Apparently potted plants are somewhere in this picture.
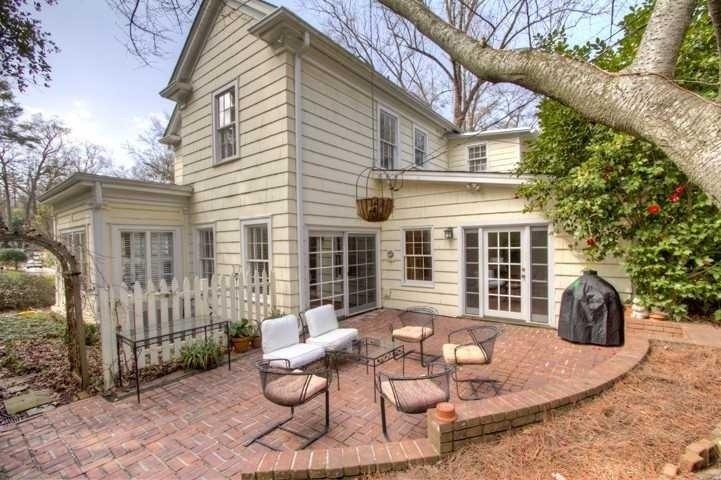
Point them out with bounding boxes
[177,335,223,372]
[229,318,264,352]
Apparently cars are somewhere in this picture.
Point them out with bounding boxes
[25,252,42,269]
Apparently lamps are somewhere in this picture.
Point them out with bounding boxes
[443,228,454,242]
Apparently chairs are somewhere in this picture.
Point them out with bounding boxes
[441,325,499,401]
[388,305,441,367]
[242,357,329,452]
[255,303,361,391]
[377,368,452,433]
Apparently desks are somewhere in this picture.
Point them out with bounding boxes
[116,315,232,402]
